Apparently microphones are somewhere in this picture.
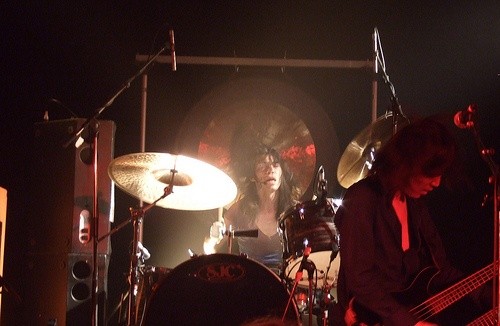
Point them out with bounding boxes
[365,30,378,74]
[295,247,311,281]
[169,29,176,71]
[454,103,477,128]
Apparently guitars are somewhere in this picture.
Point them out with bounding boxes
[347,262,500,326]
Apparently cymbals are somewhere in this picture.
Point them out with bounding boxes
[337,110,395,190]
[107,152,238,210]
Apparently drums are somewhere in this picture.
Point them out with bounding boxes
[279,197,342,288]
[136,266,175,326]
[150,252,302,326]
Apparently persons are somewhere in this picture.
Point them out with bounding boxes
[333,121,492,326]
[203,148,305,277]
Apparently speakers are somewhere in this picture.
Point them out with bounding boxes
[18,120,114,326]
